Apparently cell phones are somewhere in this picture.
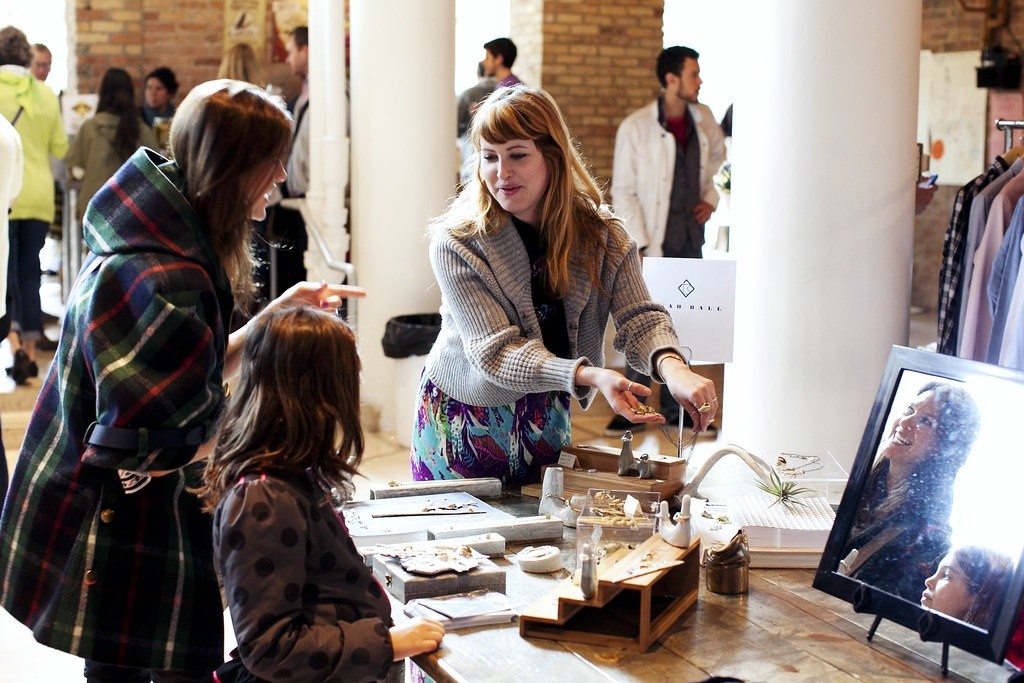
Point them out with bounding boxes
[926,174,938,186]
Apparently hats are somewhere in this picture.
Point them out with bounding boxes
[145,66,177,96]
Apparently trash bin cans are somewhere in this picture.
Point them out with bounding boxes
[387,314,442,448]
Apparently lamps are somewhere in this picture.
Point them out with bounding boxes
[642,256,738,455]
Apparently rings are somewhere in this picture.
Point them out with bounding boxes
[628,382,633,391]
[690,396,718,423]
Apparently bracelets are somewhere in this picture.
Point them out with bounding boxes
[657,356,682,383]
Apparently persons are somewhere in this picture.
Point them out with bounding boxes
[217,43,260,87]
[838,380,978,608]
[457,37,522,136]
[410,84,718,487]
[611,47,727,258]
[283,26,310,282]
[0,25,69,385]
[137,67,178,129]
[919,543,1013,633]
[19,44,60,352]
[0,112,23,521]
[0,79,368,683]
[71,66,160,226]
[187,306,444,683]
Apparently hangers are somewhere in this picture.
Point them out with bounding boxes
[1002,119,1024,166]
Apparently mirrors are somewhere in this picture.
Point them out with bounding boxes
[809,344,1024,673]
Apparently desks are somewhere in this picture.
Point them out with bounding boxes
[339,486,969,683]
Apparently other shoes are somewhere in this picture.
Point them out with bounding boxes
[10,351,31,383]
[21,334,59,350]
[5,362,38,377]
[38,311,59,324]
[662,413,717,437]
[604,415,646,435]
